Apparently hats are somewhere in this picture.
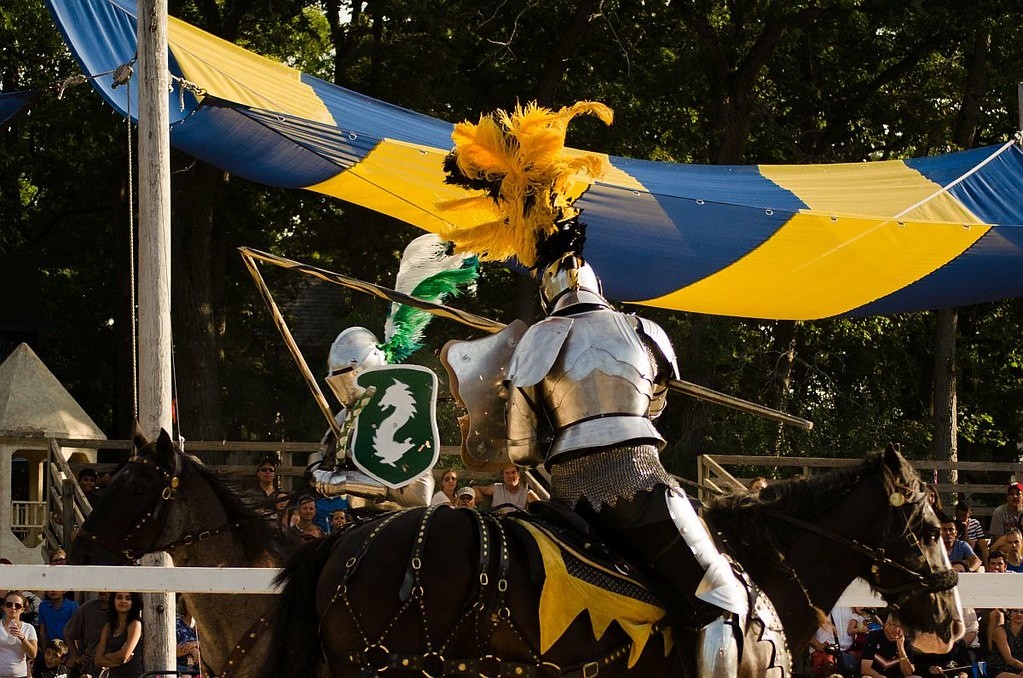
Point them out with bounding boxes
[1007,482,1023,491]
[457,487,476,498]
[259,456,275,466]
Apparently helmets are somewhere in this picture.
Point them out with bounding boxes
[326,326,390,409]
[540,255,609,316]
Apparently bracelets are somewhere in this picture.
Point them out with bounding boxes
[899,656,908,661]
[21,636,25,641]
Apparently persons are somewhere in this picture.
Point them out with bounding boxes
[305,325,435,512]
[807,483,1023,678]
[430,468,459,507]
[0,548,206,678]
[475,465,541,513]
[75,468,111,520]
[254,459,359,539]
[504,255,746,678]
[748,477,768,493]
[451,487,476,510]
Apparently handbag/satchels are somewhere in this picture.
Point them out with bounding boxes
[837,650,860,678]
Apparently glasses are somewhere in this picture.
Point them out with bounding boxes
[258,467,275,472]
[4,601,22,610]
[444,477,458,481]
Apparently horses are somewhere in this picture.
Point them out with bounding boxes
[64,420,318,678]
[255,437,966,677]
